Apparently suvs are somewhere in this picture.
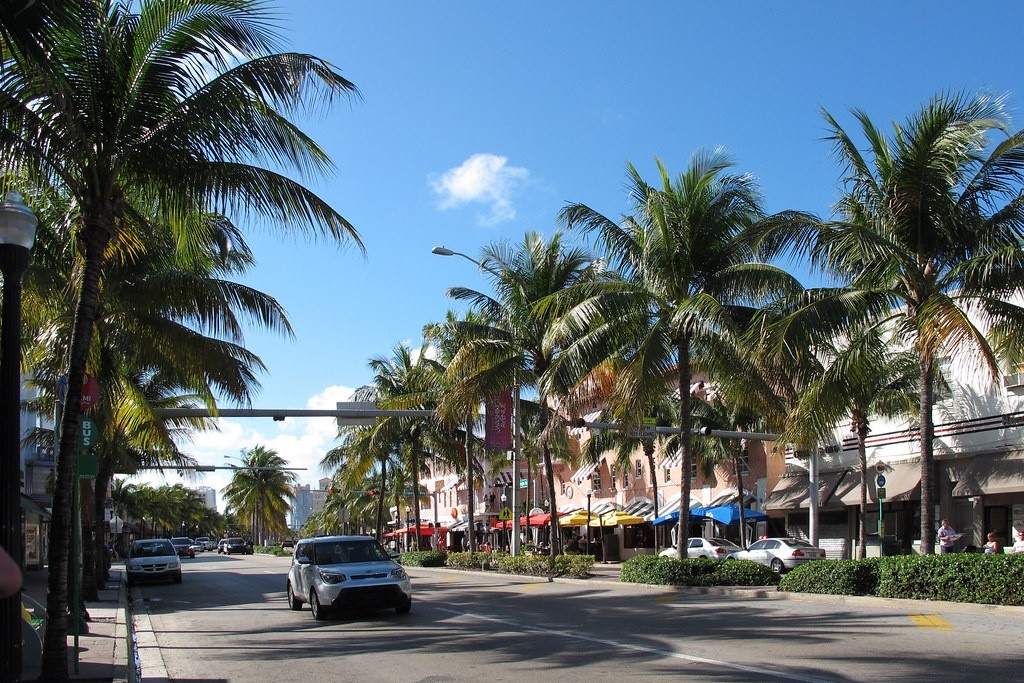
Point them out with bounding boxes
[287,535,412,621]
[168,537,196,558]
[195,536,213,551]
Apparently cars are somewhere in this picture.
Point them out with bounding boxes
[281,540,295,549]
[216,539,227,554]
[191,541,204,552]
[125,538,185,584]
[659,537,743,563]
[223,537,247,556]
[729,536,826,574]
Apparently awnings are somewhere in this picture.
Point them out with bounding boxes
[448,520,486,534]
[553,381,1024,522]
[484,471,526,489]
[19,491,52,519]
[440,475,466,493]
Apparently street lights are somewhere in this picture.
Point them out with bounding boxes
[225,456,265,547]
[432,245,522,558]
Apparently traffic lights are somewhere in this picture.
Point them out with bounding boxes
[369,490,381,495]
[328,488,342,494]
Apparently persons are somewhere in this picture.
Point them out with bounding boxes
[983,532,998,553]
[461,531,599,556]
[937,519,956,555]
[104,536,120,561]
[1011,532,1024,554]
[240,540,254,555]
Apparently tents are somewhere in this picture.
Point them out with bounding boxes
[653,506,769,547]
[383,523,449,552]
[495,510,646,562]
